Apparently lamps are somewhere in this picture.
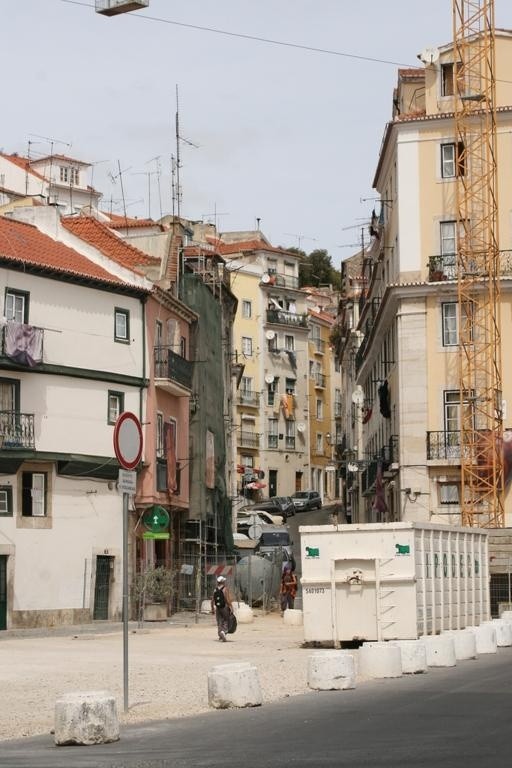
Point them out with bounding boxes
[325,432,337,446]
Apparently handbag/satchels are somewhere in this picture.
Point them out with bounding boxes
[228,614,236,633]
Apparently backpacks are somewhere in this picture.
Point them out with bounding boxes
[214,587,225,608]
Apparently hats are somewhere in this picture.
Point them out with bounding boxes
[217,575,226,583]
[284,566,291,572]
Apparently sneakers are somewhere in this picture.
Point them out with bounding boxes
[219,631,227,642]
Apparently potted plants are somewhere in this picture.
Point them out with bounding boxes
[426,256,444,281]
[143,566,175,621]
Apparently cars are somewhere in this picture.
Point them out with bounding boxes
[236,490,323,574]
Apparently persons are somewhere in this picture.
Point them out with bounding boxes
[278,563,299,617]
[210,576,237,641]
